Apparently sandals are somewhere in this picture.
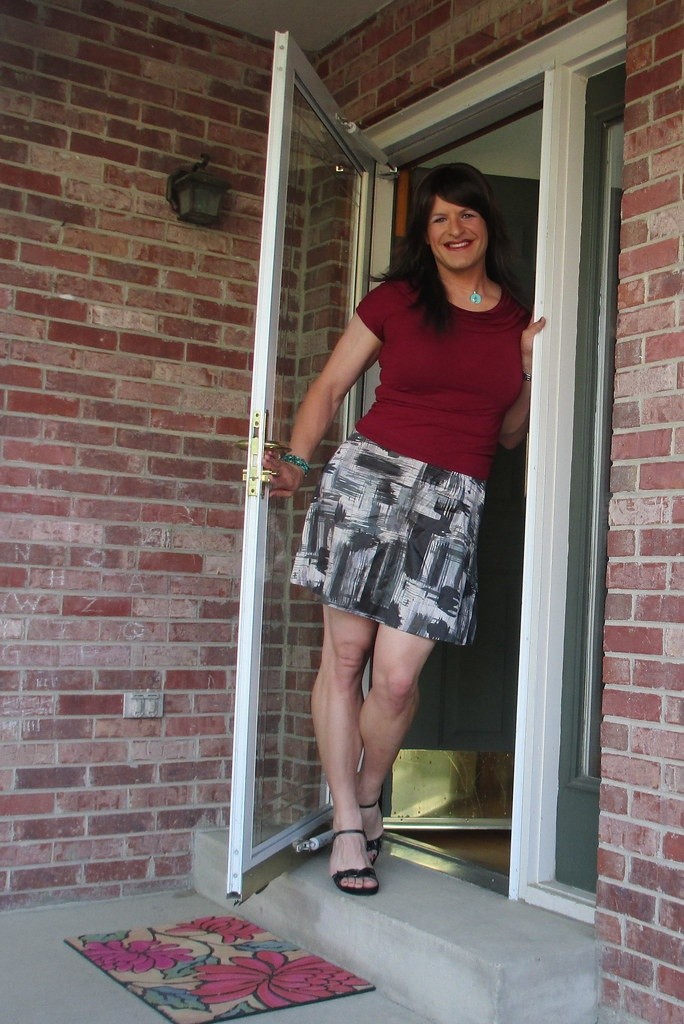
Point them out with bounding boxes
[359,801,383,863]
[331,830,379,895]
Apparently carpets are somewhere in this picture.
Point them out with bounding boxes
[64,914,376,1024]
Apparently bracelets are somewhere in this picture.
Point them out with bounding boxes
[281,455,310,477]
[521,370,531,382]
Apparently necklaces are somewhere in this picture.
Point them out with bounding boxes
[441,278,489,303]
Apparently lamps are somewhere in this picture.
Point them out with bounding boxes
[166,154,231,225]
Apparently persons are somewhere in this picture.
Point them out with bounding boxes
[262,162,546,896]
